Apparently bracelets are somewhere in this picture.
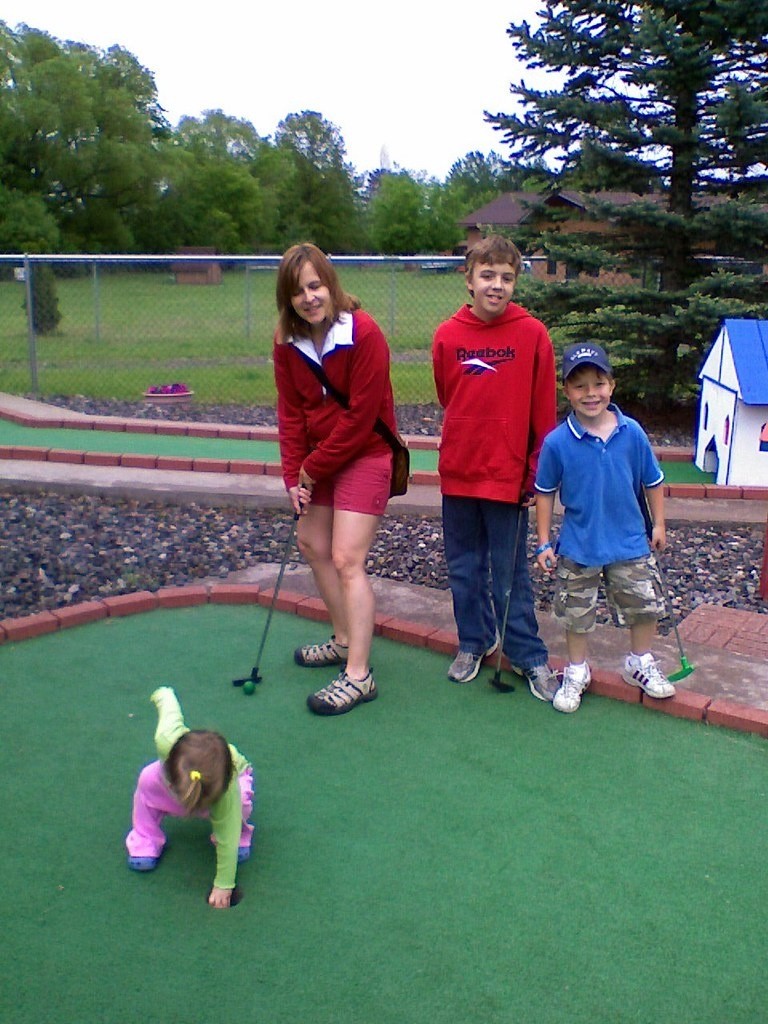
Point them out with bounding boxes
[534,542,552,557]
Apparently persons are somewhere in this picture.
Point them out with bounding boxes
[534,342,676,712]
[125,686,255,908]
[432,236,561,703]
[273,244,398,716]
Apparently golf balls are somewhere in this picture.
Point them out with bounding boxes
[242,681,257,695]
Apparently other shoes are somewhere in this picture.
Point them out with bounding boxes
[128,855,157,871]
[238,847,249,863]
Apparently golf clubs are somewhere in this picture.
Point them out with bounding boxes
[638,482,697,683]
[231,483,308,688]
[487,494,527,693]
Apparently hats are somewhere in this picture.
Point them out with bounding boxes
[563,344,612,380]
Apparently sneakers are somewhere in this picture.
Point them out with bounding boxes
[511,661,560,702]
[295,635,348,667]
[307,667,377,716]
[554,662,591,712]
[624,656,676,699]
[449,633,499,682]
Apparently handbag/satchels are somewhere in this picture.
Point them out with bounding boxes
[389,445,410,501]
